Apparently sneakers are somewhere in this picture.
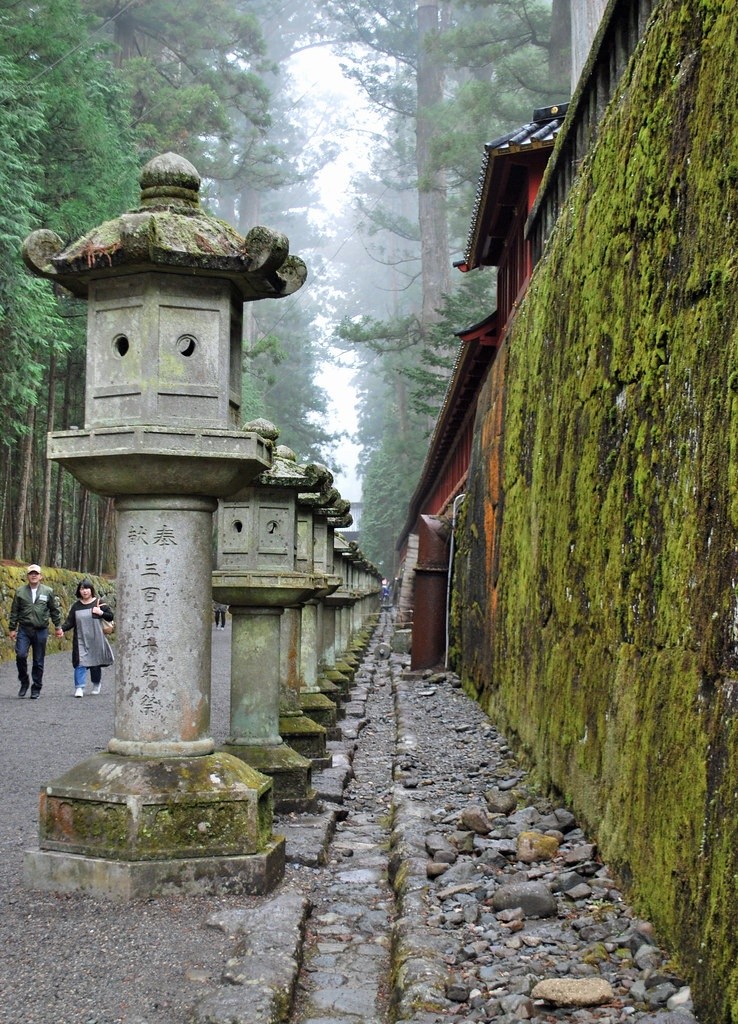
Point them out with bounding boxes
[91,683,101,694]
[75,688,83,697]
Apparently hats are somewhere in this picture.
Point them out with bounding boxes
[27,564,41,573]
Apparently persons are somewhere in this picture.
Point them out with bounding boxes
[8,564,61,699]
[211,601,227,630]
[59,578,114,697]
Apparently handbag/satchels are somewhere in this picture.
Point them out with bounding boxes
[97,599,116,634]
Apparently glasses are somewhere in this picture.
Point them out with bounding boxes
[29,572,39,575]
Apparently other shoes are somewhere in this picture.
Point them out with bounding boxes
[31,694,39,698]
[216,626,219,629]
[221,628,224,631]
[19,688,27,696]
